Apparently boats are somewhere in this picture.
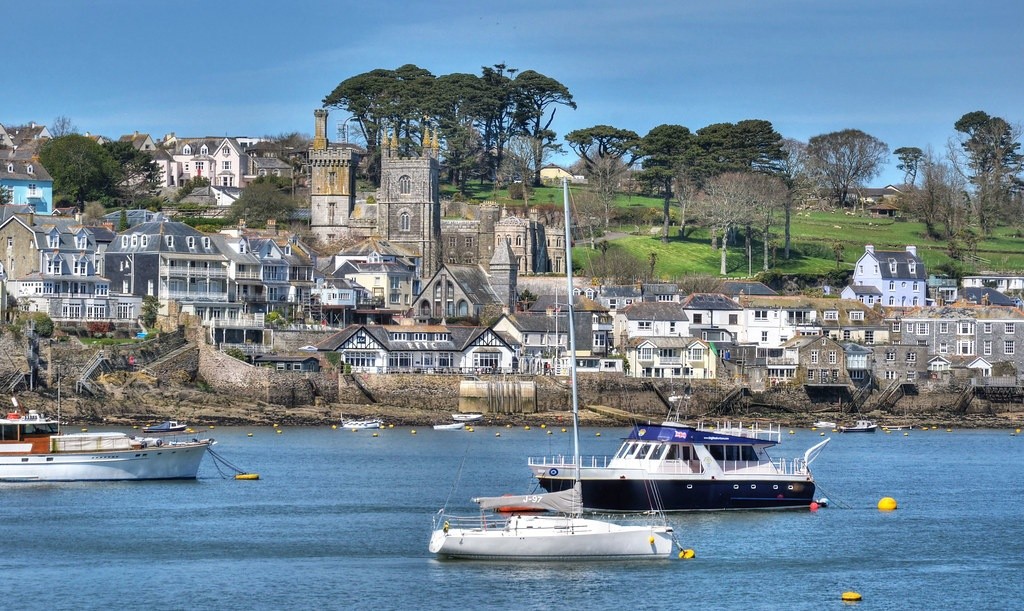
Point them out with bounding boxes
[837,420,878,433]
[813,421,836,429]
[142,420,187,433]
[0,364,214,482]
[433,423,465,429]
[341,412,384,429]
[452,414,482,420]
[235,473,259,480]
[527,406,852,515]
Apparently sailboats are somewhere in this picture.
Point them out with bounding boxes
[428,176,683,560]
